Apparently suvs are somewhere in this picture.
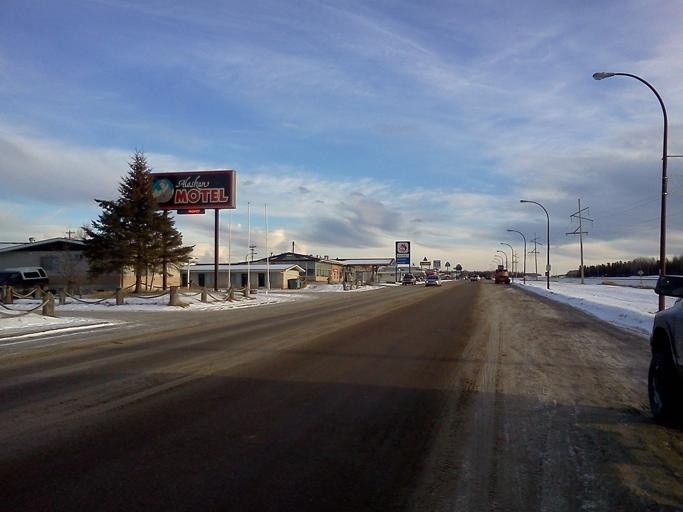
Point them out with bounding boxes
[402,273,416,286]
[0,265,48,302]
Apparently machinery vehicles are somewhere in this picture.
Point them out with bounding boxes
[494,265,509,285]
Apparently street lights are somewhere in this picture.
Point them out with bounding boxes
[591,70,668,312]
[496,250,507,270]
[500,242,513,282]
[506,229,526,284]
[491,254,503,266]
[519,199,550,289]
[244,252,257,262]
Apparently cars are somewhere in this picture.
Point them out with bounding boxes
[414,268,492,282]
[425,274,441,287]
[647,274,682,427]
